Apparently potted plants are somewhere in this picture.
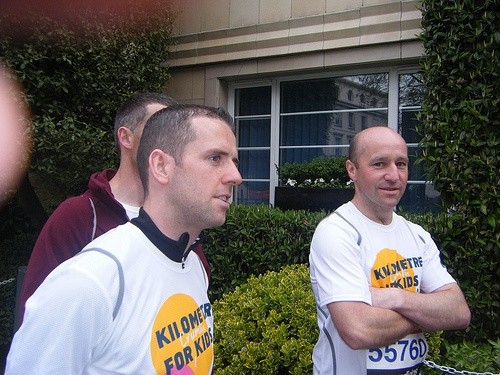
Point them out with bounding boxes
[274,154,356,214]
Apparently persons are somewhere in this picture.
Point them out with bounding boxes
[15,91,212,339]
[3,102,243,375]
[307,126,470,375]
